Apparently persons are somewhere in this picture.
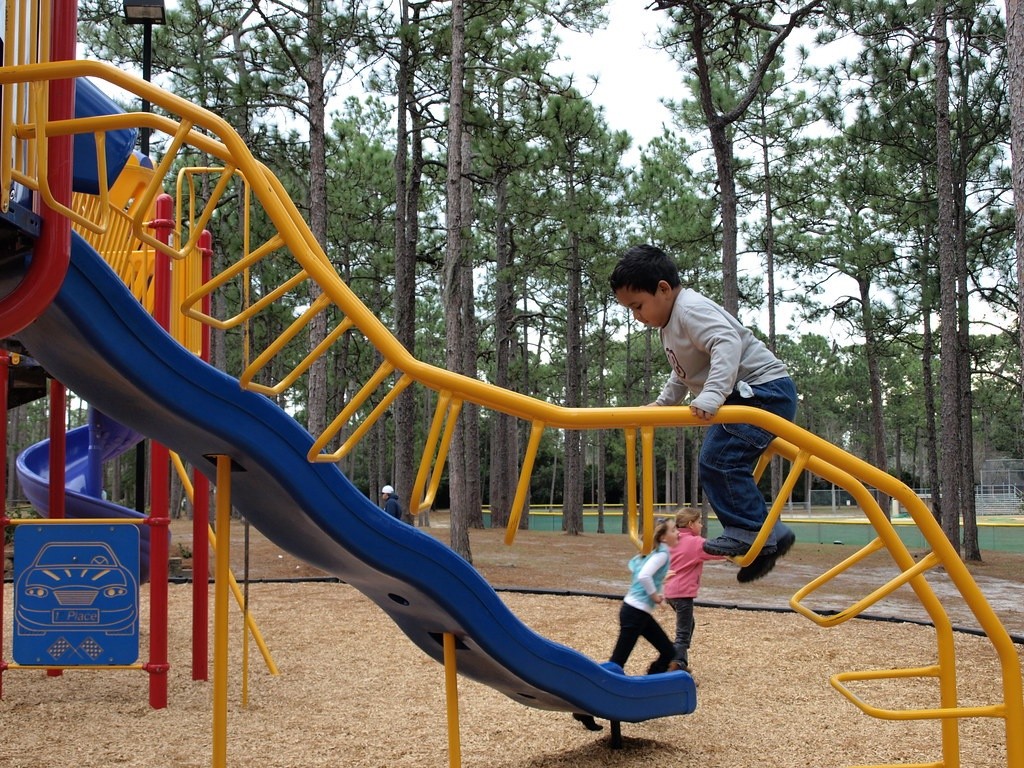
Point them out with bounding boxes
[608,244,797,583]
[662,506,726,673]
[381,485,402,521]
[572,518,680,732]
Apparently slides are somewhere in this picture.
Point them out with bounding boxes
[14,387,172,586]
[0,194,695,719]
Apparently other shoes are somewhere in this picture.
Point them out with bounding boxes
[573,713,603,731]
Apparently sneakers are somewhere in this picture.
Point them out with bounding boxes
[702,535,778,557]
[737,530,796,583]
